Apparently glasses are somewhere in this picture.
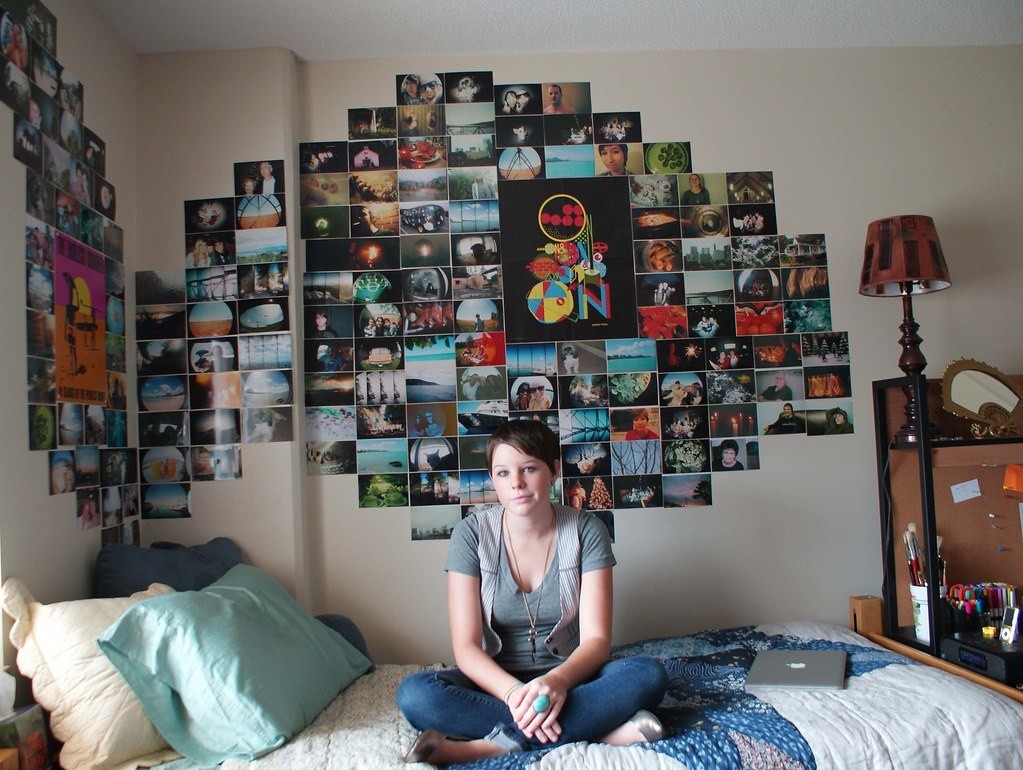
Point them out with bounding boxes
[419,81,443,93]
[530,386,544,393]
[516,93,530,99]
[521,389,530,394]
[426,415,431,418]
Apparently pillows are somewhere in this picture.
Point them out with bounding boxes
[0,576,184,770]
[314,614,375,675]
[93,537,245,599]
[98,559,374,768]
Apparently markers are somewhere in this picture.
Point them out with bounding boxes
[948,582,1019,632]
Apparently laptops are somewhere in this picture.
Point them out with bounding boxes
[744,650,848,690]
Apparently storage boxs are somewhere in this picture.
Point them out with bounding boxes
[0,704,52,770]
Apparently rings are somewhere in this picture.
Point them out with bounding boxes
[533,695,550,713]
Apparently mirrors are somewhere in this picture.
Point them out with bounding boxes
[940,356,1023,439]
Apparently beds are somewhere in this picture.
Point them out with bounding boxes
[12,595,1023,770]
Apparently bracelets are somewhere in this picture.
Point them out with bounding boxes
[504,682,524,705]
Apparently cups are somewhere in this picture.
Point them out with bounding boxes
[909,583,947,645]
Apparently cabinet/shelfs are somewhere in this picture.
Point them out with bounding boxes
[871,374,1023,658]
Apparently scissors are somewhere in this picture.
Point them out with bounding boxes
[950,584,975,603]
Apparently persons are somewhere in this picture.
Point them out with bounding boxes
[186,237,232,267]
[757,371,792,402]
[597,144,637,176]
[543,83,577,114]
[825,407,854,435]
[737,212,764,232]
[716,349,740,370]
[681,174,710,205]
[318,345,354,371]
[599,120,626,141]
[653,281,682,306]
[513,380,553,410]
[763,403,806,434]
[309,312,338,338]
[697,316,719,338]
[625,408,660,439]
[713,439,744,471]
[393,419,669,764]
[242,161,279,194]
[363,317,400,337]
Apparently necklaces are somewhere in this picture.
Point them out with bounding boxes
[503,509,558,649]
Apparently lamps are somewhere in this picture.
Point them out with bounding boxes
[857,215,953,446]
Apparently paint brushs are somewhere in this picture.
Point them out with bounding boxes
[902,522,946,586]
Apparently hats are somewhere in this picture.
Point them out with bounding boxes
[837,410,847,419]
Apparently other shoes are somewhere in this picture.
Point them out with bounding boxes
[403,728,478,764]
[629,708,664,744]
